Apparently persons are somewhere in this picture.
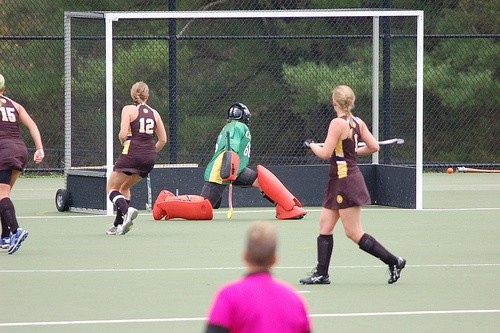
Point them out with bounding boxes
[299,85,406,284]
[0,74,45,254]
[206,220,311,333]
[153,103,307,221]
[105,81,167,235]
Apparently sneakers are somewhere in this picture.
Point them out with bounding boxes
[387,256,407,284]
[0,237,10,250]
[300,268,331,284]
[8,228,28,254]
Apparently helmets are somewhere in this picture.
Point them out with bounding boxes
[229,103,250,121]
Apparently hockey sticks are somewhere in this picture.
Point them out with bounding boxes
[226,131,234,218]
[146,173,152,213]
[313,139,405,148]
[456,167,500,173]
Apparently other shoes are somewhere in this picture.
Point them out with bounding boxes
[119,207,138,235]
[106,226,117,235]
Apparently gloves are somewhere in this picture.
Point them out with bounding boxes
[303,139,317,149]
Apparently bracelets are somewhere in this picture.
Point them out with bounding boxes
[36,148,42,150]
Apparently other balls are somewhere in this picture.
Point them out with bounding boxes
[447,168,453,174]
